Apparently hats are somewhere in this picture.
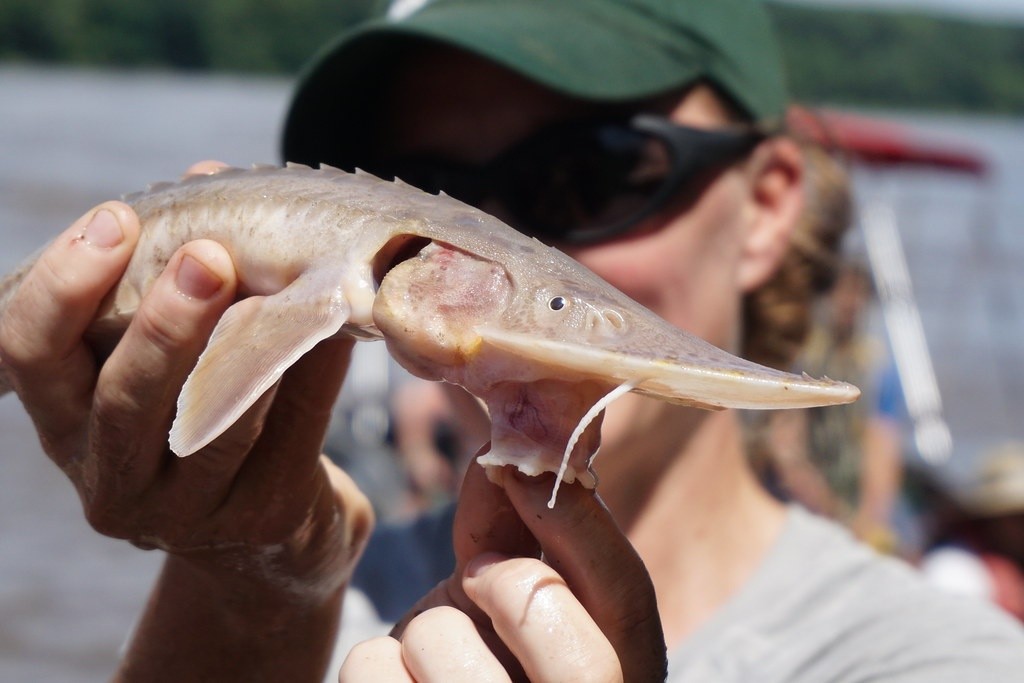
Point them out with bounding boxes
[276,0,795,156]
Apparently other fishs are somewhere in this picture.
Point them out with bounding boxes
[0,161,861,509]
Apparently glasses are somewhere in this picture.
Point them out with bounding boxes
[354,107,774,253]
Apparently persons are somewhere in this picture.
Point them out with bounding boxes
[760,260,926,567]
[321,340,500,594]
[0,0,1022,683]
